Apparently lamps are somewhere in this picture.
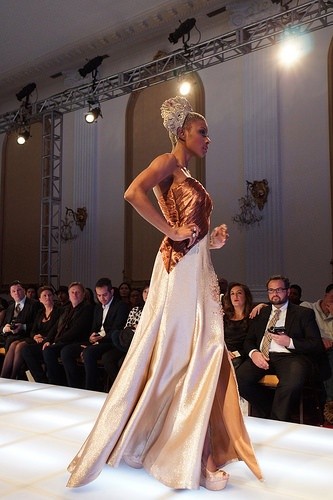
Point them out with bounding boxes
[169,18,197,51]
[231,179,270,228]
[85,104,101,123]
[16,129,30,145]
[16,83,37,104]
[53,207,87,246]
[78,56,104,82]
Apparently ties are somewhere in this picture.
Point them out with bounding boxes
[261,309,281,359]
[14,304,20,317]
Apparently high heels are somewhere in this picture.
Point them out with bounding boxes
[199,463,229,491]
[122,454,142,469]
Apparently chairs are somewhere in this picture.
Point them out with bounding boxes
[260,374,304,423]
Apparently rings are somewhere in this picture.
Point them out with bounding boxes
[193,227,197,232]
[192,232,196,238]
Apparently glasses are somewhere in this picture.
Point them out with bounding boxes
[267,287,287,294]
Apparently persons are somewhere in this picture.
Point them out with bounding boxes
[66,96,268,493]
[0,275,333,429]
